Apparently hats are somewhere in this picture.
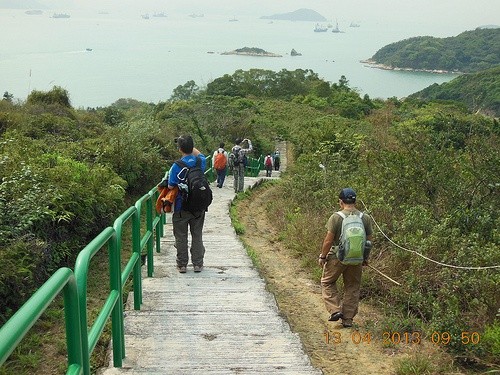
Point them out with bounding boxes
[235,138,243,145]
[338,189,356,203]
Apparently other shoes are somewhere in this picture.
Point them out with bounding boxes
[176,267,187,273]
[342,321,354,327]
[194,265,203,272]
[327,312,343,321]
[217,183,222,188]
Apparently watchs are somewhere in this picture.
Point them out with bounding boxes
[319,254,326,259]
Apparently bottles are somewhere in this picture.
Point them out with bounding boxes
[181,189,189,200]
[338,242,344,249]
[366,239,372,247]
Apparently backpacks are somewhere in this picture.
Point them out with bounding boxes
[228,148,243,171]
[266,157,271,167]
[175,156,213,217]
[334,211,367,266]
[214,150,227,170]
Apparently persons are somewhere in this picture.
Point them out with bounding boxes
[273,152,280,171]
[167,134,206,273]
[264,153,274,177]
[232,138,252,193]
[212,142,228,188]
[319,188,373,327]
